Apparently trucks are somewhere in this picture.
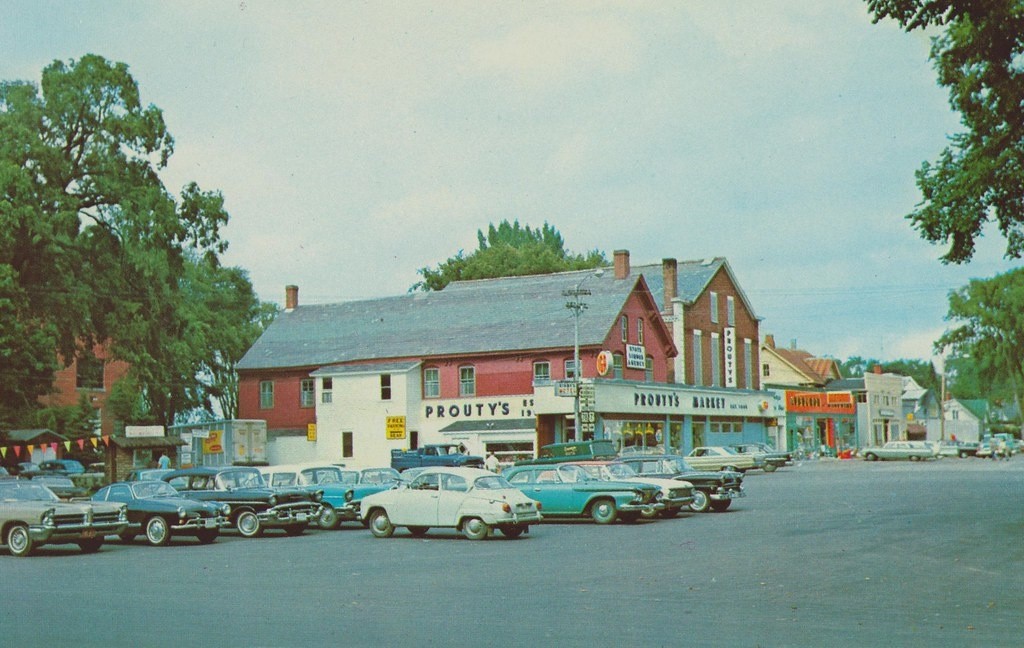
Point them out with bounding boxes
[391,445,484,470]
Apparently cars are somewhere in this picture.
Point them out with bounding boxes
[731,444,791,472]
[89,481,232,547]
[502,467,664,526]
[0,479,130,556]
[613,455,745,513]
[579,462,696,519]
[862,441,933,462]
[340,467,408,491]
[684,447,767,474]
[0,459,103,482]
[355,468,543,540]
[299,466,389,529]
[936,433,1024,459]
[160,467,324,537]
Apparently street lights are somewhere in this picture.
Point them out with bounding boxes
[562,268,606,443]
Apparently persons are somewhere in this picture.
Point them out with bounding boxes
[157,452,171,469]
[485,449,501,474]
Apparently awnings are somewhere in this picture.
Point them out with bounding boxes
[439,418,534,436]
